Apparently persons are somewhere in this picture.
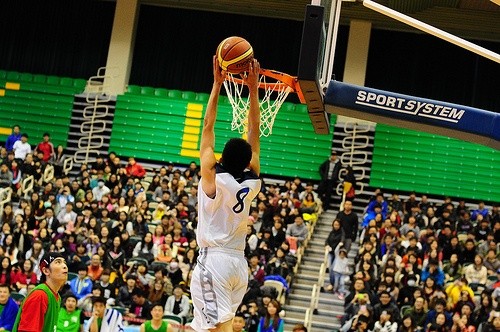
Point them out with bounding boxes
[0,125,500,332]
[190,55,262,332]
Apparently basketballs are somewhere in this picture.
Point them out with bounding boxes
[217,35,254,74]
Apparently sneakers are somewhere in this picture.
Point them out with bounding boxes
[330,290,337,294]
[338,295,344,299]
